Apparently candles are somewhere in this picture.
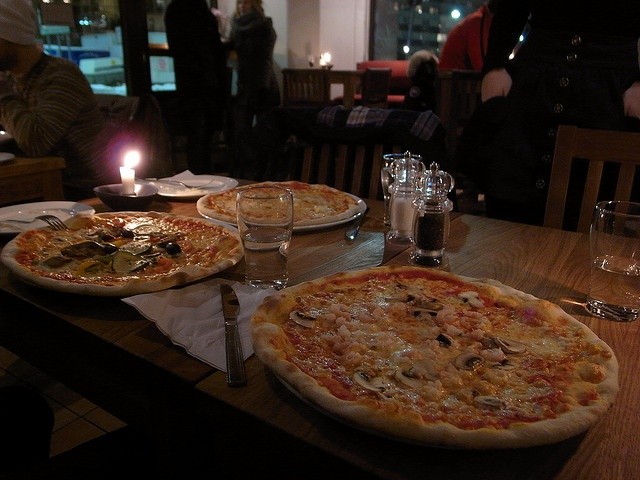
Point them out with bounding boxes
[117,150,141,197]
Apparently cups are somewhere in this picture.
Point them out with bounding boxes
[236,186,293,292]
[584,200,640,321]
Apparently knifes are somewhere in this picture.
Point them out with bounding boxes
[343,217,364,241]
[219,282,248,390]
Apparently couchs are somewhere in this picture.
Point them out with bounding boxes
[352,60,411,102]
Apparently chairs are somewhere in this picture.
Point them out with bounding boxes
[541,125,640,240]
[282,68,331,100]
[301,110,430,200]
[92,92,139,128]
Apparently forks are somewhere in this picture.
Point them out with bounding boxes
[2,214,73,234]
[141,176,218,189]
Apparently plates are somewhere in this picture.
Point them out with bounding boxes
[1,199,96,233]
[147,174,239,197]
[93,176,172,211]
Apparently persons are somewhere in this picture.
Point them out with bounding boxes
[481,0,640,226]
[438,0,501,74]
[164,0,245,175]
[0,0,122,202]
[230,1,279,127]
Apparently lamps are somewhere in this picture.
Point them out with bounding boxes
[326,52,334,69]
[319,56,326,70]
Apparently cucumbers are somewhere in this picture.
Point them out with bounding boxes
[111,241,162,273]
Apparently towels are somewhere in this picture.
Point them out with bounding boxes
[120,278,278,373]
